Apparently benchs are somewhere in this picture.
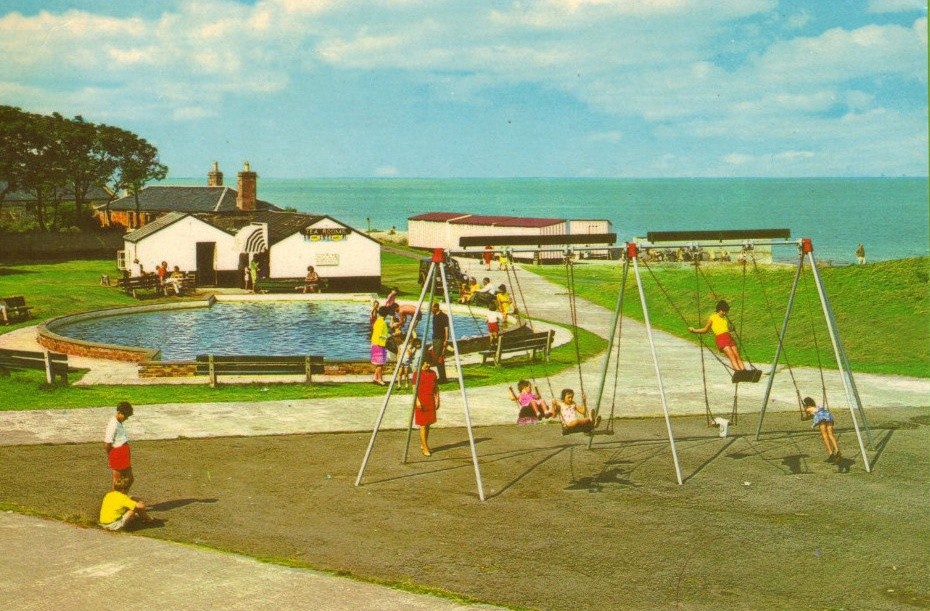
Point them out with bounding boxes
[194,353,325,385]
[159,273,196,294]
[0,348,67,388]
[0,295,34,320]
[479,329,555,367]
[255,277,329,293]
[119,275,158,299]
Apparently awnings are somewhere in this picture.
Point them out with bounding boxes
[234,225,266,253]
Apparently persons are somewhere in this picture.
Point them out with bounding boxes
[370,287,422,390]
[856,245,866,264]
[646,248,703,261]
[412,355,440,455]
[508,380,552,420]
[104,402,134,490]
[484,246,507,270]
[244,258,261,290]
[0,298,10,325]
[462,276,512,344]
[804,397,841,463]
[721,250,746,262]
[551,388,602,427]
[432,303,449,384]
[687,300,745,370]
[131,259,183,296]
[100,476,157,530]
[304,266,320,293]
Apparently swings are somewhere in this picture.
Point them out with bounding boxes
[451,257,627,438]
[640,248,836,429]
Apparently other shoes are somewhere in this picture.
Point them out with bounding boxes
[372,379,378,383]
[405,385,409,389]
[377,380,385,386]
[835,451,843,463]
[536,413,543,420]
[827,453,835,462]
[145,517,157,523]
[591,409,596,421]
[544,412,552,418]
[420,446,433,453]
[594,416,601,428]
[422,449,430,457]
[395,386,401,390]
